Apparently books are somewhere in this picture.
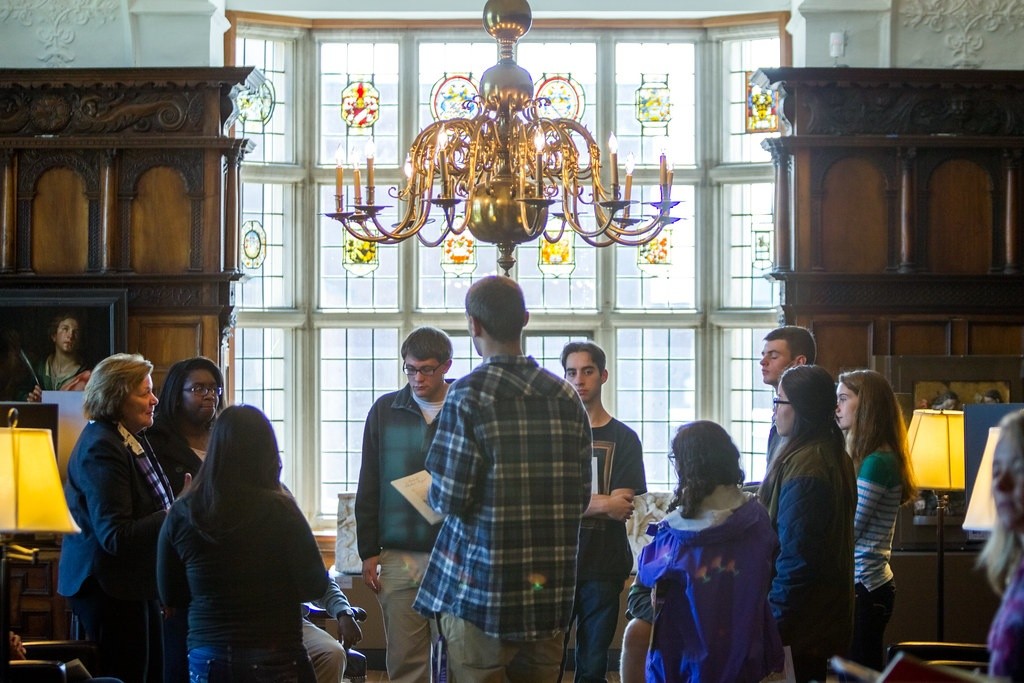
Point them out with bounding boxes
[390,471,446,525]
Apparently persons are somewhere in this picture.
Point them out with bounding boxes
[759,326,816,468]
[834,370,917,683]
[56,354,194,683]
[411,277,592,683]
[757,365,858,683]
[147,357,229,498]
[301,570,363,683]
[619,570,654,683]
[354,326,458,683]
[638,421,785,683]
[8,632,126,683]
[977,410,1024,683]
[560,342,647,683]
[157,405,330,683]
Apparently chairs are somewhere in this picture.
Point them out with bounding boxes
[300,601,368,683]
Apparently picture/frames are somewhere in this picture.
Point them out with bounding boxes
[0,287,131,403]
[872,353,1024,553]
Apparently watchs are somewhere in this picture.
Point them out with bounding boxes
[336,609,356,621]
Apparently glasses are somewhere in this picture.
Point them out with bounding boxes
[773,398,791,410]
[668,452,676,462]
[402,362,444,376]
[182,386,222,396]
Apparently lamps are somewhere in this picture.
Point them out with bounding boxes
[0,408,83,683]
[317,0,685,276]
[904,407,964,640]
